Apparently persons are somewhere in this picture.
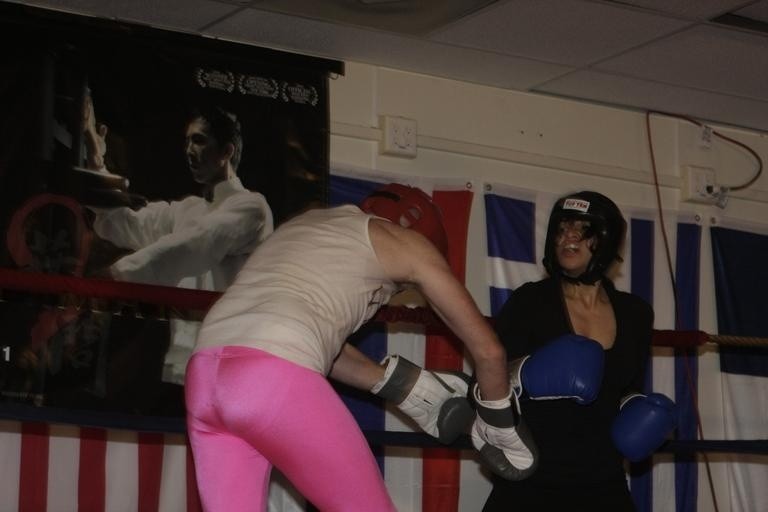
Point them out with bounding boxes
[183,181,541,512]
[461,191,679,511]
[15,76,275,420]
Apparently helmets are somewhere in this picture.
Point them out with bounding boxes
[544,191,627,283]
[362,183,448,263]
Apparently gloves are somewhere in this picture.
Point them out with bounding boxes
[611,392,682,459]
[509,335,605,407]
[469,382,541,482]
[372,352,475,450]
